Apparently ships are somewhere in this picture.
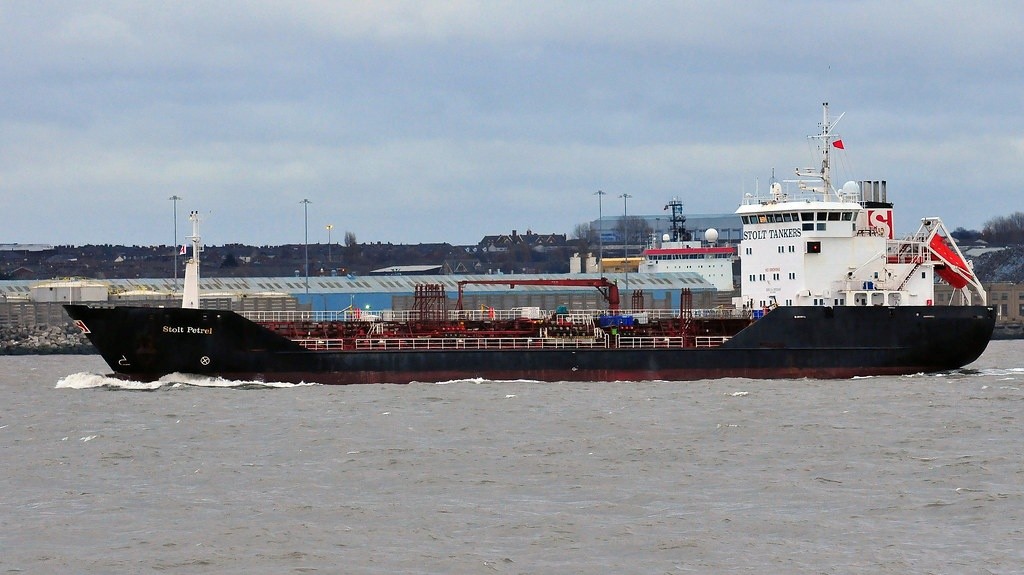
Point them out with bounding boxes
[59,102,998,384]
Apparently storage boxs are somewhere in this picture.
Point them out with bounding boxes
[600,314,634,330]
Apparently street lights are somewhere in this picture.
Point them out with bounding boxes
[298,199,313,296]
[618,193,633,290]
[593,190,606,279]
[168,195,182,292]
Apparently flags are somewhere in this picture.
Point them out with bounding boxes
[833,140,844,149]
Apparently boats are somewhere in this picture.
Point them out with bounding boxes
[926,234,972,292]
